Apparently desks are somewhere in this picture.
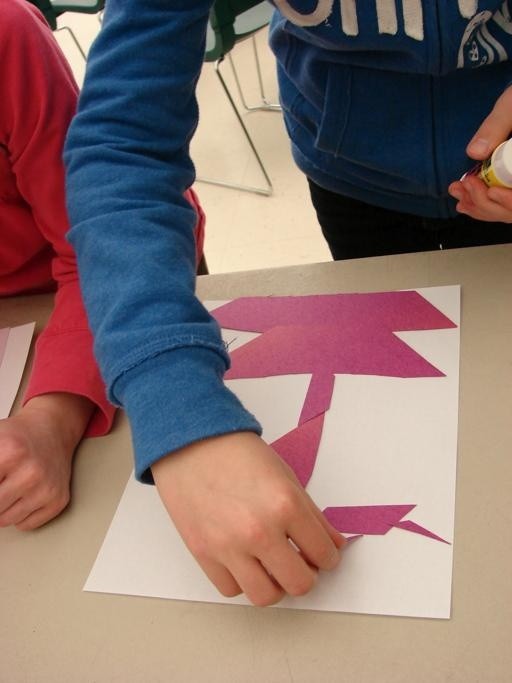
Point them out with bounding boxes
[2,241,509,683]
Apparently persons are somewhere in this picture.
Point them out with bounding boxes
[60,1,511,609]
[1,1,209,533]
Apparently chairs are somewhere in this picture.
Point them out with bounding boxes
[189,0,283,196]
